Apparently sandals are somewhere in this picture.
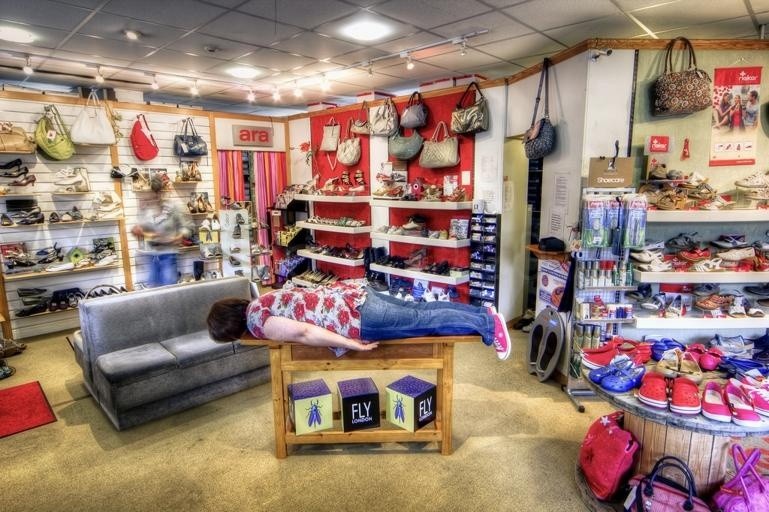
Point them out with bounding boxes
[175,162,272,284]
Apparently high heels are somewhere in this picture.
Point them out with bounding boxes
[0,155,118,272]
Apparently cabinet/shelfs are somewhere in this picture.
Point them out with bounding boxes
[631,209,769,330]
[0,190,133,320]
[562,255,637,413]
[292,193,471,288]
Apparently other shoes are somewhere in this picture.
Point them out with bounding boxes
[625,282,769,319]
[578,328,769,428]
[637,164,717,211]
[630,230,769,273]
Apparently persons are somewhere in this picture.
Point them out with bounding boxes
[712,92,732,129]
[131,175,192,287]
[746,90,760,129]
[730,94,744,130]
[207,282,512,362]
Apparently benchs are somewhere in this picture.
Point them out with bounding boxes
[73,275,271,431]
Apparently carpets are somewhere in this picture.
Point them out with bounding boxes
[0,380,57,439]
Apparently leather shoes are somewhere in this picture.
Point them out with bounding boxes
[16,286,128,317]
[297,173,464,302]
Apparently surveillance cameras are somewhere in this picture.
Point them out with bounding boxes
[592,49,613,59]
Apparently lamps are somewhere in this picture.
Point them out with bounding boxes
[272,43,467,101]
[23,55,256,103]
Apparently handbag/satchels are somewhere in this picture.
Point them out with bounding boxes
[652,66,712,116]
[320,82,489,171]
[276,184,304,279]
[580,410,768,512]
[522,118,555,159]
[0,88,208,160]
[0,337,26,356]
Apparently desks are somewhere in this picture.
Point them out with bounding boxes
[239,333,482,459]
[574,361,769,511]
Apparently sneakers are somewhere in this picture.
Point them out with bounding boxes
[735,170,769,188]
[488,306,512,360]
